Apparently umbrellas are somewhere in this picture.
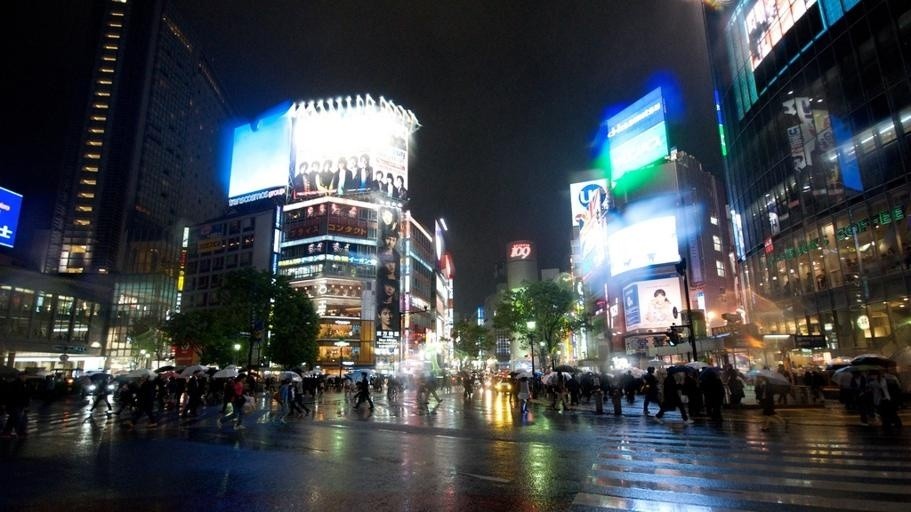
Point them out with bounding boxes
[849,354,898,370]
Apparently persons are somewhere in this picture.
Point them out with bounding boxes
[1,362,911,441]
[293,153,407,331]
[647,289,674,321]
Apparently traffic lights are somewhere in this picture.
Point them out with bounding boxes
[234,344,241,352]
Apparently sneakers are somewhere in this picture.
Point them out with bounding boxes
[217,419,246,431]
[684,419,695,425]
[281,409,311,424]
[654,416,663,424]
[353,405,374,409]
[424,399,443,404]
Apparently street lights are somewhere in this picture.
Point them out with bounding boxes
[526,320,539,400]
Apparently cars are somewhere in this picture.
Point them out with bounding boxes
[88,374,114,395]
[451,368,513,396]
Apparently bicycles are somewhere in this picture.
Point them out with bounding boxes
[388,383,399,400]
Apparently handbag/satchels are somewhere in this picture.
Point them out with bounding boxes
[274,393,282,401]
